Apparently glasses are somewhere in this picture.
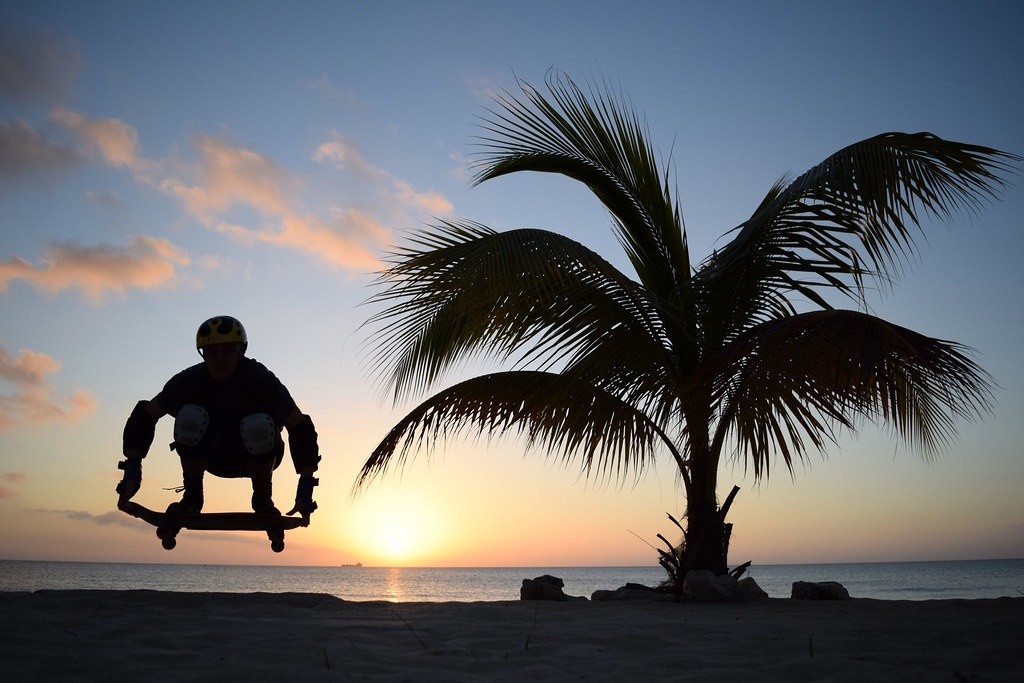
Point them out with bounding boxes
[204,346,237,360]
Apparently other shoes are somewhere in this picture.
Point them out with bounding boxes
[167,496,202,516]
[253,496,280,522]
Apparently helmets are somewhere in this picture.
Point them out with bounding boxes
[196,317,243,347]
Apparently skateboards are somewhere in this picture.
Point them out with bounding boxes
[117,500,313,552]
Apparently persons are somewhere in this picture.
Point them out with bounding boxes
[116,316,322,527]
